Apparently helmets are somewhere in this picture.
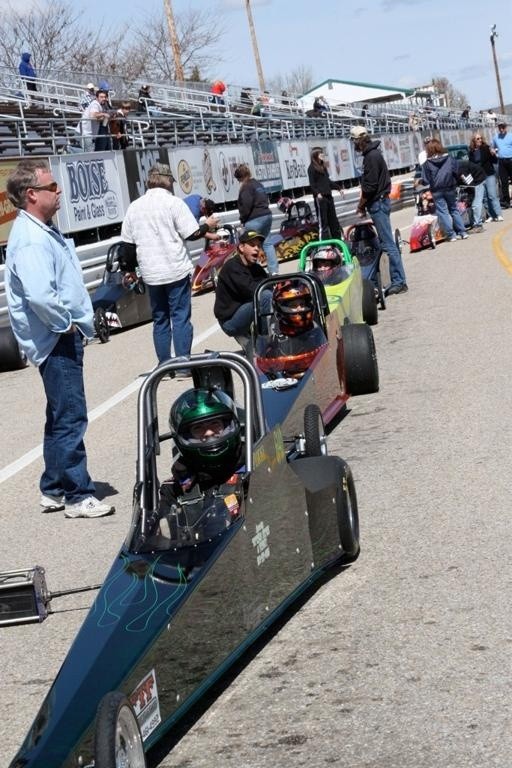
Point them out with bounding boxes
[273,278,315,338]
[214,229,233,248]
[278,197,292,214]
[169,387,242,476]
[311,245,341,282]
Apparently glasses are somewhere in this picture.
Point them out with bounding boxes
[30,182,58,192]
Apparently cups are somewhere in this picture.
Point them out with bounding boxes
[101,116,109,127]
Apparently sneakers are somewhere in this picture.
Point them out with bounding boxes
[64,493,117,519]
[387,284,408,294]
[447,214,504,243]
[162,369,192,380]
[40,492,66,510]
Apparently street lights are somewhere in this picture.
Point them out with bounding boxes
[489,20,507,116]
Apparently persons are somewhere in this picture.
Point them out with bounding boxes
[362,104,370,116]
[415,121,512,242]
[93,82,128,151]
[233,166,278,275]
[276,197,316,238]
[408,105,439,131]
[259,279,328,359]
[209,81,331,143]
[118,269,138,289]
[461,105,498,129]
[350,127,407,292]
[80,82,97,112]
[212,227,279,362]
[19,52,38,99]
[116,162,223,381]
[308,146,342,240]
[137,84,156,117]
[4,158,116,520]
[311,248,348,284]
[61,90,112,154]
[133,385,247,543]
[111,102,131,150]
[182,195,221,241]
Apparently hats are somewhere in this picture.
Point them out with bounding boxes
[348,126,369,140]
[238,229,266,245]
[204,198,215,214]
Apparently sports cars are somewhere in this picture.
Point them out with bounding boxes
[0,326,28,371]
[6,350,361,766]
[342,219,403,288]
[275,202,320,263]
[87,240,155,333]
[395,145,500,259]
[225,274,375,462]
[282,239,380,339]
[192,223,245,292]
